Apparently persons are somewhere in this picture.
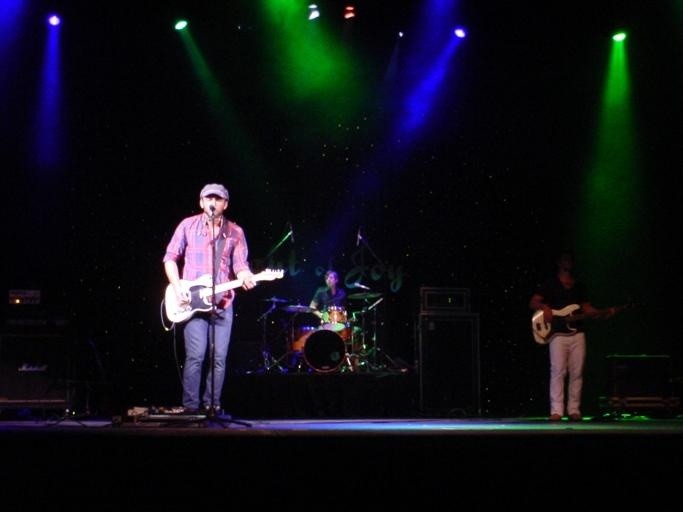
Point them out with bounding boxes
[527,246,618,420]
[162,183,257,422]
[309,270,348,326]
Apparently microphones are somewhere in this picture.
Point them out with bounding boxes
[209,205,216,220]
[354,225,362,247]
[287,222,297,245]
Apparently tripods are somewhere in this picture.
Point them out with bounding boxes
[156,230,253,429]
[244,327,288,377]
[362,322,399,371]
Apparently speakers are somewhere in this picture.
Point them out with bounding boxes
[419,311,482,418]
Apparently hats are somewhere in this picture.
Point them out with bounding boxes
[200,184,229,200]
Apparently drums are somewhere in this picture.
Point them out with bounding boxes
[303,331,347,372]
[327,306,347,322]
[322,322,349,340]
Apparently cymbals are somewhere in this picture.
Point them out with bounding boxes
[284,305,310,312]
[263,298,288,302]
[347,293,380,299]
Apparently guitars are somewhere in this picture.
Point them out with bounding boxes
[164,268,284,323]
[531,302,624,344]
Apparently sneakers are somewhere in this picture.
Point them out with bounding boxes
[570,415,581,421]
[550,414,561,420]
